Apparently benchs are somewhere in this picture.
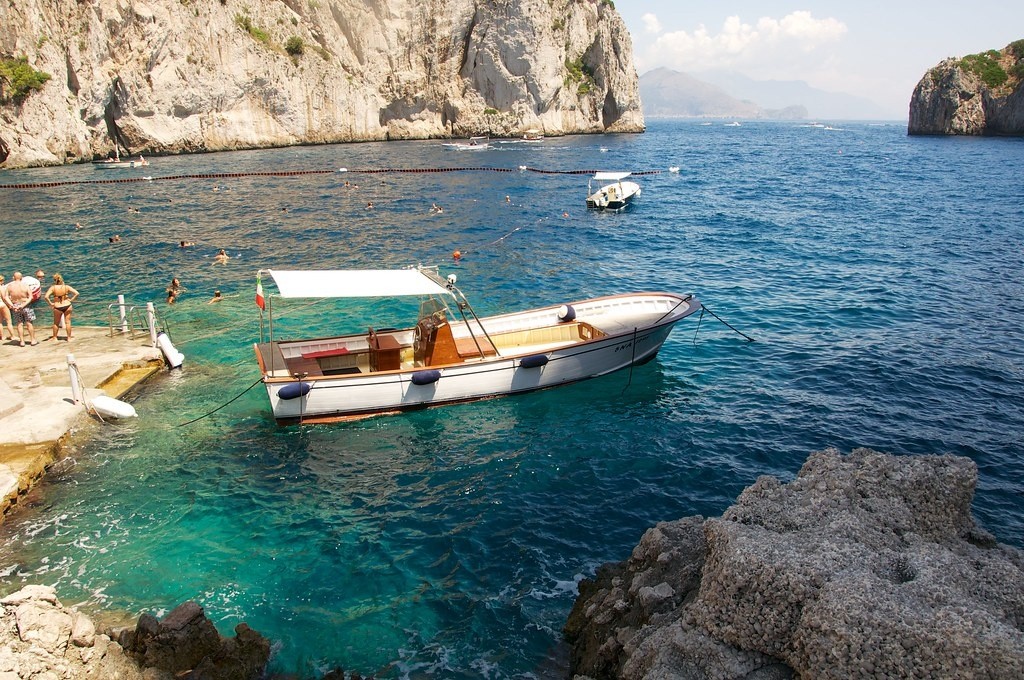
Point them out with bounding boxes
[285,343,411,377]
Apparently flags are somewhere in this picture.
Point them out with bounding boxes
[255,272,265,311]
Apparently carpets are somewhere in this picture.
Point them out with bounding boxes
[322,367,362,376]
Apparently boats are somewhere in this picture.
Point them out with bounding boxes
[585,170,641,210]
[254,267,702,422]
[89,135,143,168]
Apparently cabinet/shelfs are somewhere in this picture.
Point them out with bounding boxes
[364,326,401,373]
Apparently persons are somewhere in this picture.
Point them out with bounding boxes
[380,180,387,186]
[108,234,121,244]
[365,201,373,211]
[76,223,83,228]
[179,240,195,247]
[44,273,80,342]
[344,181,358,189]
[280,207,288,214]
[165,277,188,306]
[206,290,225,305]
[506,196,510,202]
[140,155,146,163]
[107,156,118,162]
[428,202,443,213]
[128,206,140,213]
[212,249,231,266]
[213,186,220,191]
[0,272,39,347]
